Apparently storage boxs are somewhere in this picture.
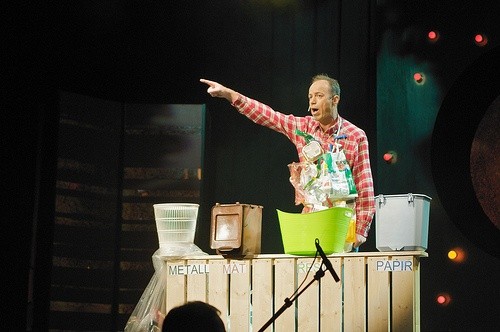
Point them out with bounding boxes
[275,208,354,254]
[210,201,263,258]
[374,193,432,251]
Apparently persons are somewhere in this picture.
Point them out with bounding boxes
[162,300,227,332]
[199,73,375,252]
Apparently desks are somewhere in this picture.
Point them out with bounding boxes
[163,251,429,332]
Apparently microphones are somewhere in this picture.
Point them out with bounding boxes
[308,106,310,111]
[315,242,340,282]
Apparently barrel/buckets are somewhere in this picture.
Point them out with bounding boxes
[276,206,353,255]
[373,193,433,251]
[155,204,200,257]
